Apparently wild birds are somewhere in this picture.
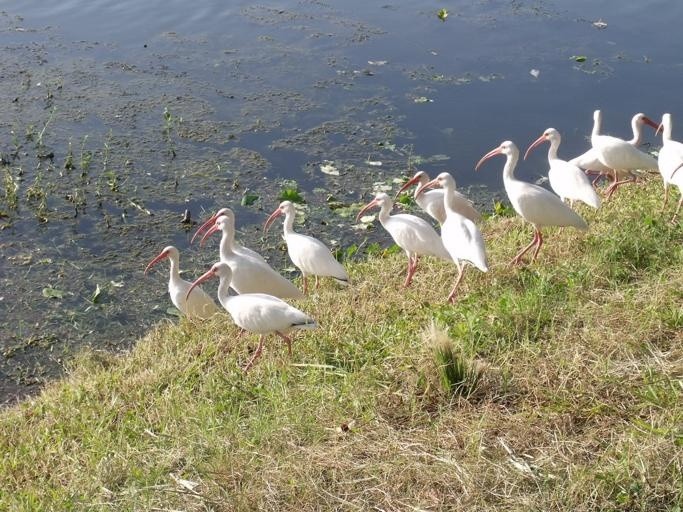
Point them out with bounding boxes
[523,127,601,235]
[190,207,270,267]
[355,170,490,306]
[198,215,307,340]
[262,200,349,295]
[567,110,683,223]
[185,261,319,375]
[143,245,222,321]
[474,140,589,266]
